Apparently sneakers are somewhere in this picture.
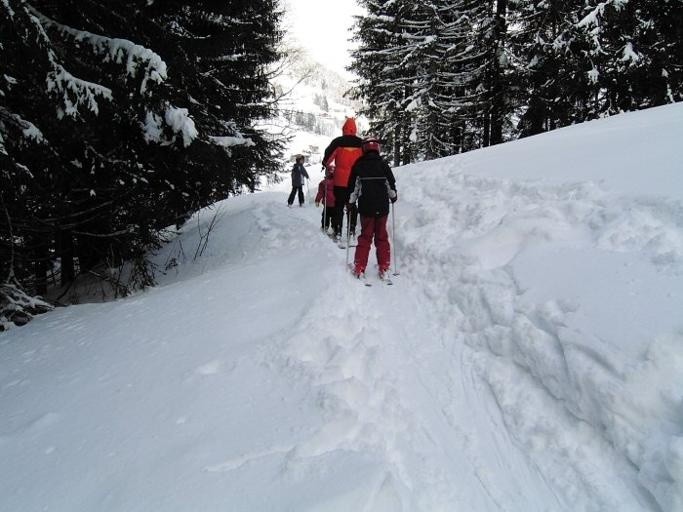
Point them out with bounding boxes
[353,264,365,276]
[379,268,387,277]
[350,232,358,240]
[332,232,342,241]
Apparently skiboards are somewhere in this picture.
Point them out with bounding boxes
[323,228,395,286]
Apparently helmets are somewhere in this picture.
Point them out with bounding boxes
[327,164,335,176]
[362,137,381,153]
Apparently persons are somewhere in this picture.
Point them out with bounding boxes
[321,117,363,243]
[313,165,335,232]
[286,154,309,206]
[342,137,398,280]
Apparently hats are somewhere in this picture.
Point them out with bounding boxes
[343,120,356,132]
[296,155,304,164]
